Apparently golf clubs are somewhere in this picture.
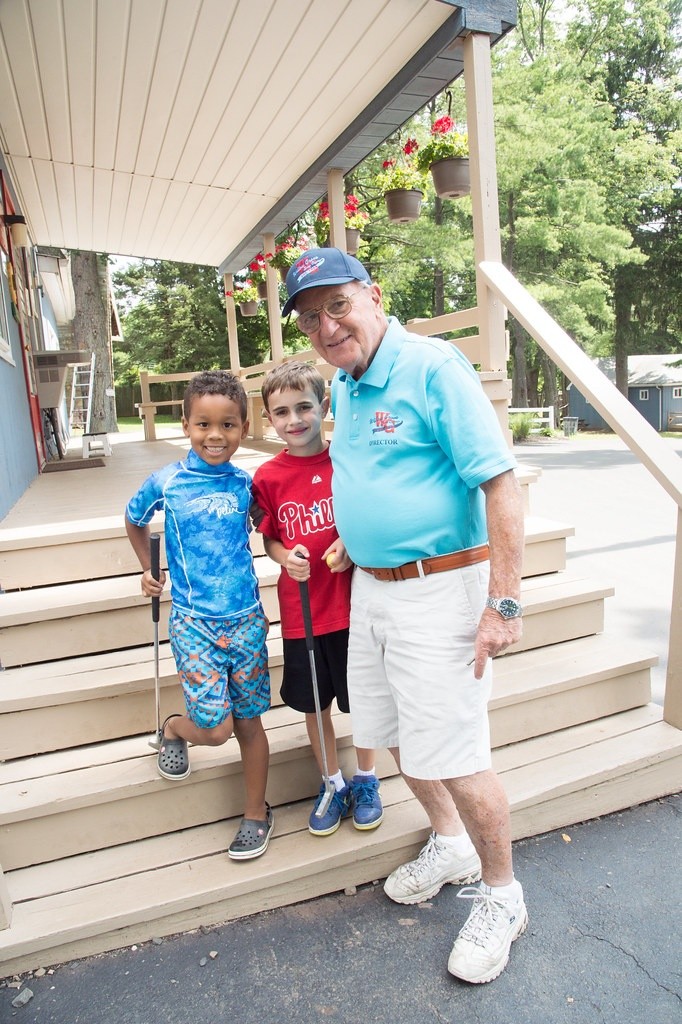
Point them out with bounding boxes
[295,551,334,819]
[149,532,165,750]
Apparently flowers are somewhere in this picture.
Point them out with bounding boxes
[416,116,470,179]
[225,284,258,306]
[247,253,267,288]
[313,194,372,247]
[265,235,310,270]
[372,138,430,202]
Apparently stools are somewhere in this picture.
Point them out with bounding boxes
[82,431,114,459]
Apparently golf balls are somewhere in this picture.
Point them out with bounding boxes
[326,553,337,566]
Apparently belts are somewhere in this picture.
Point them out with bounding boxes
[359,544,489,582]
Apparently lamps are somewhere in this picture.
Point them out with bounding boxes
[3,214,28,247]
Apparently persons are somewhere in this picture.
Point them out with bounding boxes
[281,247,529,983]
[252,362,386,837]
[124,372,276,863]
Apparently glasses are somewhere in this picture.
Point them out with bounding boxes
[293,287,366,335]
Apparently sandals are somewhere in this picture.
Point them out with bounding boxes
[229,799,275,860]
[157,714,191,781]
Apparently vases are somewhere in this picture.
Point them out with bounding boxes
[257,281,268,298]
[240,301,258,317]
[428,157,470,199]
[345,227,361,256]
[279,267,290,282]
[383,187,424,224]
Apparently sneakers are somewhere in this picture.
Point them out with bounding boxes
[448,887,539,985]
[384,835,482,905]
[349,775,385,830]
[309,777,350,834]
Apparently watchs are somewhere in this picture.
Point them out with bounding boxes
[485,597,523,620]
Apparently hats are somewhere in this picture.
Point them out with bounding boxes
[281,247,373,317]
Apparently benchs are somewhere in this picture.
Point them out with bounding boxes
[667,410,682,431]
[246,370,509,442]
